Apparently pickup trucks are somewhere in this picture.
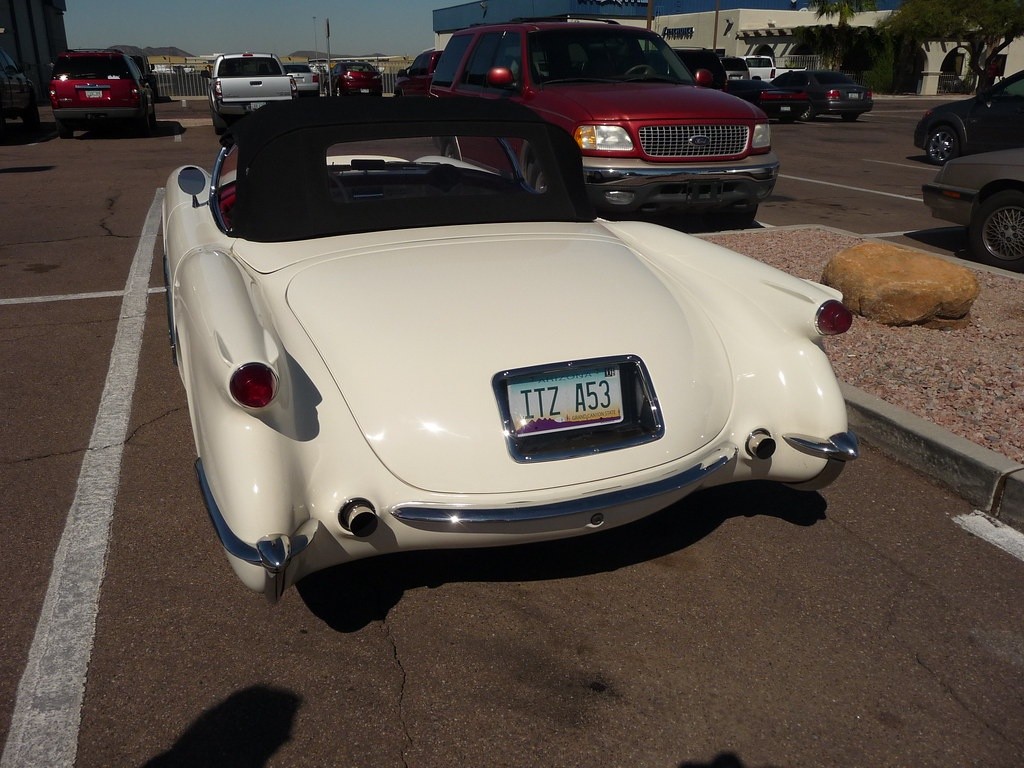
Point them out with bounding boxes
[201,52,293,135]
[738,56,806,84]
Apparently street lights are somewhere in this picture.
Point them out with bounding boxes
[312,16,332,96]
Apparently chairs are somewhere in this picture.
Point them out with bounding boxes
[584,47,616,77]
[222,62,241,76]
[258,63,272,75]
[344,65,347,71]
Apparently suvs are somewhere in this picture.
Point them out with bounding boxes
[48,49,156,139]
[128,53,158,103]
[922,148,1024,275]
[642,48,727,91]
[393,48,444,98]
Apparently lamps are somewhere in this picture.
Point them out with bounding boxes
[723,16,735,25]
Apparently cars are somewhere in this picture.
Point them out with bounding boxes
[307,64,321,75]
[430,15,780,229]
[0,48,41,133]
[768,70,874,122]
[717,56,751,80]
[159,94,860,595]
[727,80,810,123]
[324,62,383,96]
[282,62,320,97]
[914,69,1024,165]
